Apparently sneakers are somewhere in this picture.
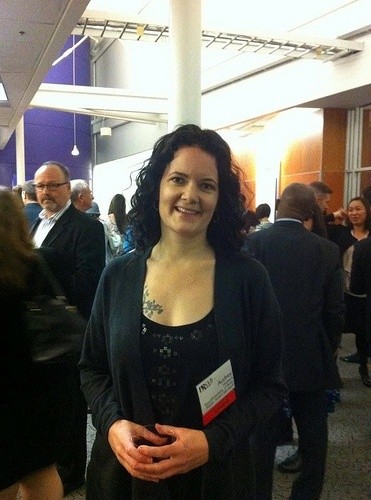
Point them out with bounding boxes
[278,450,305,474]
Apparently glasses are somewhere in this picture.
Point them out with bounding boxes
[31,181,70,190]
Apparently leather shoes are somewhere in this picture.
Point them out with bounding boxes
[341,351,361,364]
[357,365,371,387]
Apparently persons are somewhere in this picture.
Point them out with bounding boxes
[0,185,65,498]
[66,179,93,216]
[239,206,260,237]
[339,194,370,387]
[101,193,128,264]
[303,183,341,241]
[21,162,106,495]
[252,201,274,232]
[242,183,345,498]
[74,123,289,499]
[19,179,44,233]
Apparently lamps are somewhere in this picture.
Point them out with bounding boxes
[100,115,112,137]
[71,34,80,156]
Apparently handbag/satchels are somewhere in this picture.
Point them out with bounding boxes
[33,298,94,395]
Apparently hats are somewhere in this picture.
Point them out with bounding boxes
[87,202,101,215]
